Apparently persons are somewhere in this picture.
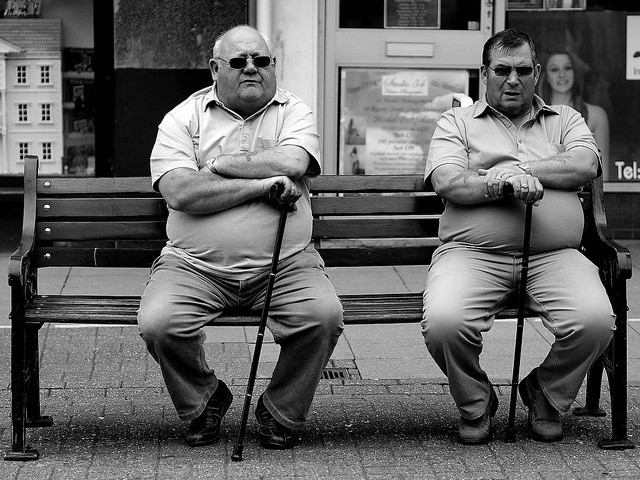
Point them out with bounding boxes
[135,24,346,450]
[537,44,610,182]
[417,27,618,443]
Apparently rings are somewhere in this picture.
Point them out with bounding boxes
[495,175,500,180]
[291,186,297,192]
[519,183,529,188]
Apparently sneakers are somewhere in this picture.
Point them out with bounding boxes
[256,393,295,449]
[519,368,562,441]
[460,381,499,445]
[185,380,232,446]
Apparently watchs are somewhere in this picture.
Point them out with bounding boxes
[205,157,217,175]
[517,162,532,175]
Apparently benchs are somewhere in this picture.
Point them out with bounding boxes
[5,149,635,458]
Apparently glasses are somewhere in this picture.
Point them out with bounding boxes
[487,65,533,76]
[219,54,271,69]
